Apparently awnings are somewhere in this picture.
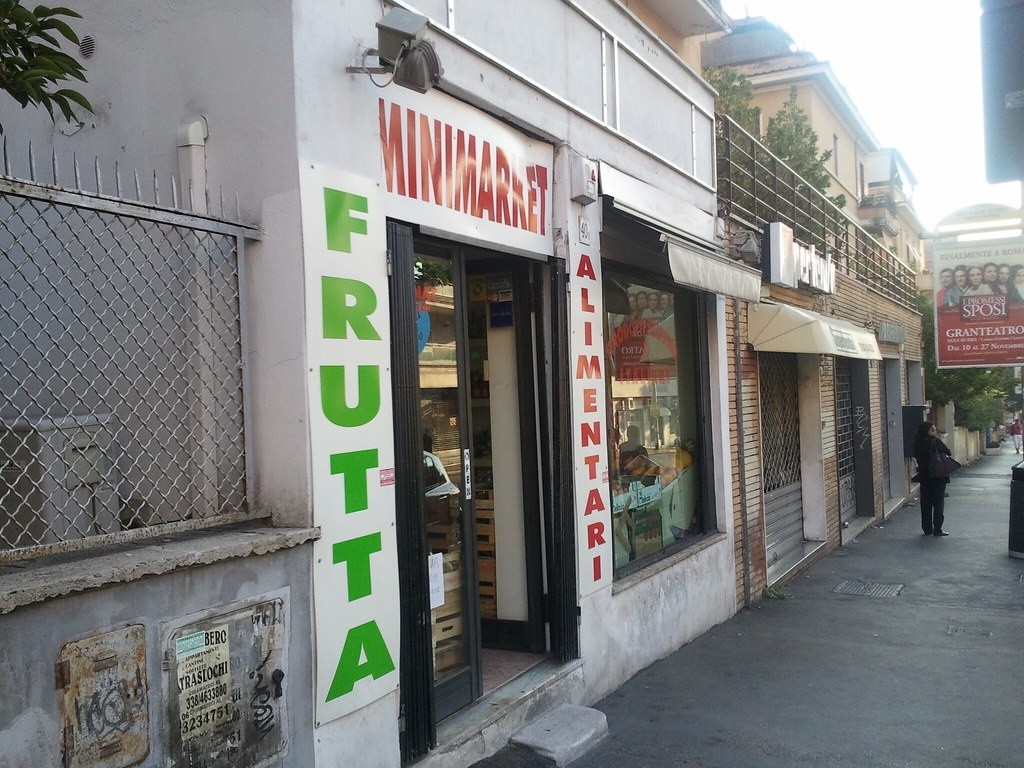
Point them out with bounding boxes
[748,302,882,361]
[620,214,762,304]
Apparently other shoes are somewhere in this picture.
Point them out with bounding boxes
[925,529,934,535]
[933,531,949,535]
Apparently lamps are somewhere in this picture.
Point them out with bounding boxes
[344,37,445,95]
[723,237,761,265]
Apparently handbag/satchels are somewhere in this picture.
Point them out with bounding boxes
[931,438,961,477]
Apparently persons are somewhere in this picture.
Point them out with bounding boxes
[1011,419,1022,454]
[938,263,1024,306]
[914,422,952,535]
[619,426,649,546]
